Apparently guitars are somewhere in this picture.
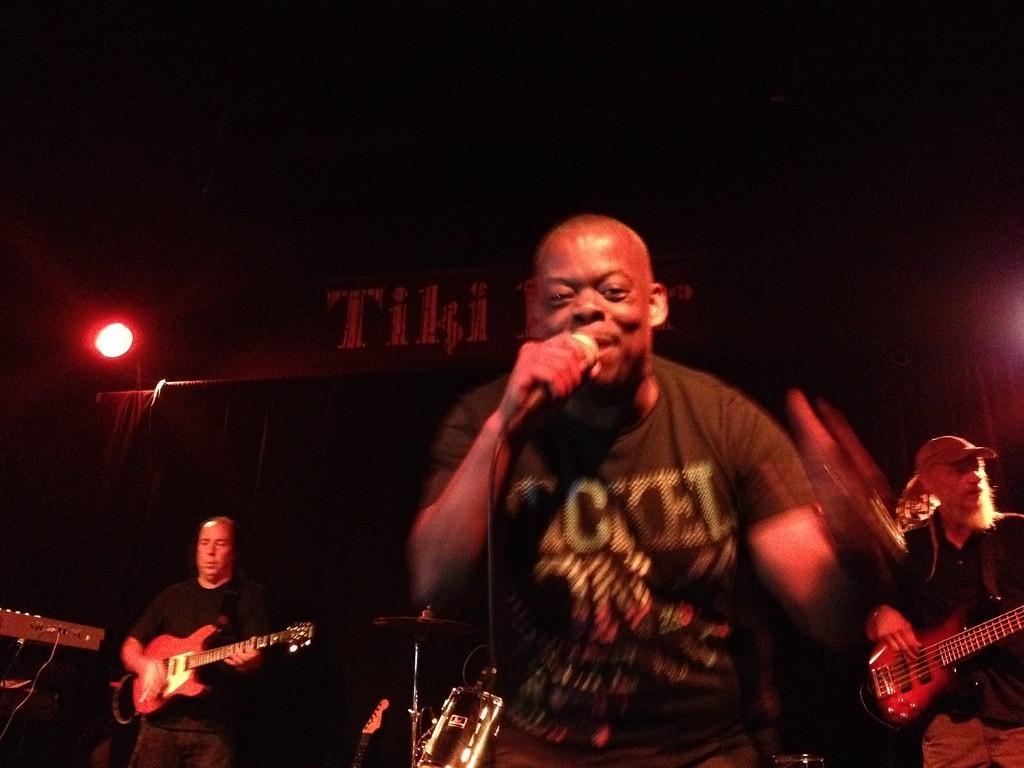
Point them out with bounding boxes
[865,600,1024,732]
[350,698,391,768]
[128,616,316,719]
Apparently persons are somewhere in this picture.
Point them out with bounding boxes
[406,212,904,768]
[118,514,282,768]
[883,432,1024,768]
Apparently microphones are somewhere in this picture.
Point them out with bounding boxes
[501,334,598,441]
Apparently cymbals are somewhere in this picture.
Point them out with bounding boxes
[370,610,481,639]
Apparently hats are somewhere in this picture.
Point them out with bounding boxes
[914,436,996,474]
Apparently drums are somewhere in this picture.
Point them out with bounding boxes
[412,683,507,768]
